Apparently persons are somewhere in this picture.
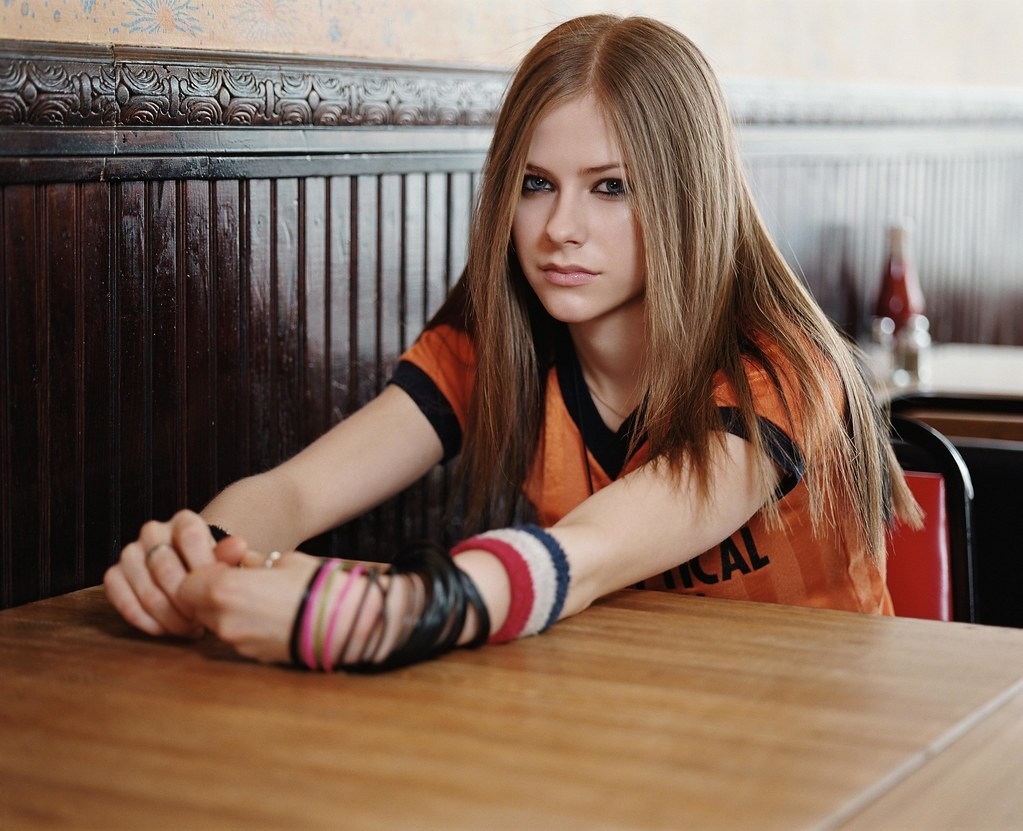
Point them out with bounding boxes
[107,16,892,671]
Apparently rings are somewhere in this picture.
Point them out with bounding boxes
[265,552,281,567]
[147,543,172,558]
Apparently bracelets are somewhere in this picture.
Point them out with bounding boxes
[451,525,570,643]
[209,524,230,544]
[287,555,490,676]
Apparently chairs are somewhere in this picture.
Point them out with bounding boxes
[884,416,976,623]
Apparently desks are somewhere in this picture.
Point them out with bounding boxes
[894,342,1023,452]
[0,557,1022,831]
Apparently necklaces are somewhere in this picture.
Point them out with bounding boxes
[588,386,627,420]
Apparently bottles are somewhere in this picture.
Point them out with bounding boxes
[872,226,926,391]
